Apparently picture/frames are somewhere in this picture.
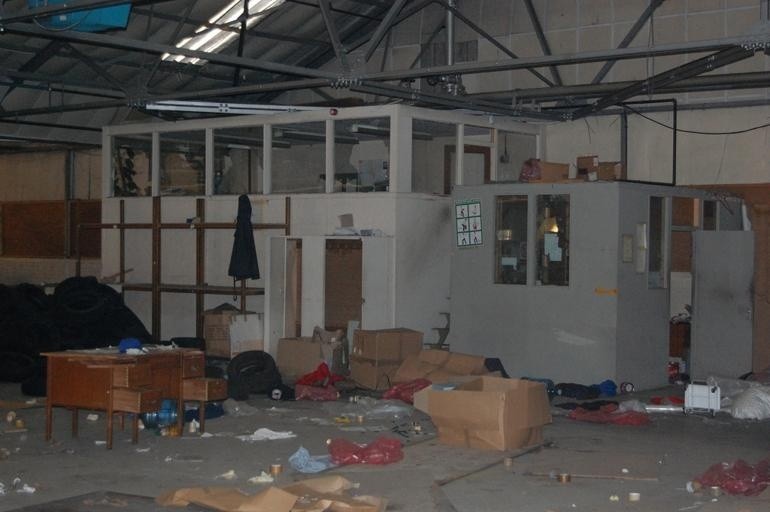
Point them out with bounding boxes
[623,234,634,263]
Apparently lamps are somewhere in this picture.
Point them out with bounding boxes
[274,127,359,144]
[350,123,433,141]
[495,131,517,182]
[160,0,288,67]
[214,134,291,148]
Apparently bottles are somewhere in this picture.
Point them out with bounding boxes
[6,411,16,422]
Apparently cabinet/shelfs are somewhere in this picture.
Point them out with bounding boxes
[39,346,228,449]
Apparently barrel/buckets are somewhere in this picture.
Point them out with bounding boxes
[144,398,177,436]
[503,238,520,271]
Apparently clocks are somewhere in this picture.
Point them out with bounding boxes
[620,382,635,396]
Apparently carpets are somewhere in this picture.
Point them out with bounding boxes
[0,490,226,512]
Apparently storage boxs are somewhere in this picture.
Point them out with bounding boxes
[528,160,570,183]
[202,310,264,360]
[276,328,553,453]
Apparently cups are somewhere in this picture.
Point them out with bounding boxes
[189,422,197,433]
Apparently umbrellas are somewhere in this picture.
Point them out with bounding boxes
[228,195,263,316]
